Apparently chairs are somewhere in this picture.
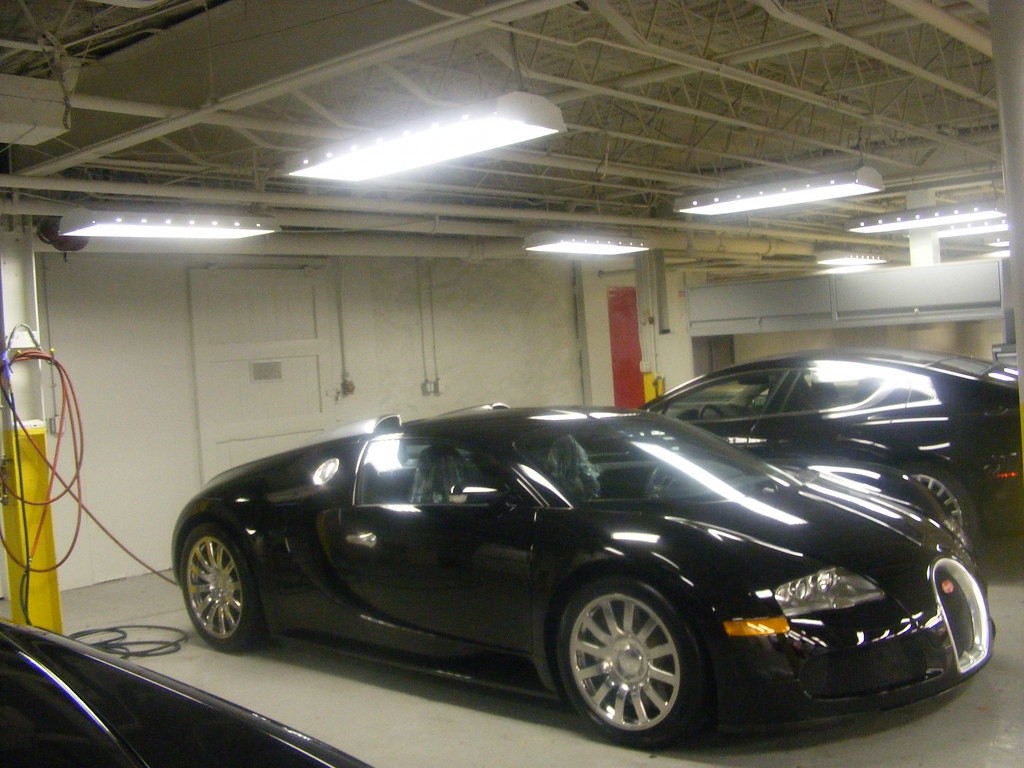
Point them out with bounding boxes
[540,434,600,504]
[409,446,473,504]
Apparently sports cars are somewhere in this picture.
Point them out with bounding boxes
[169,402,995,748]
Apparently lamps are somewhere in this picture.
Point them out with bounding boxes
[523,227,649,254]
[283,23,569,183]
[53,206,281,243]
[671,167,1010,272]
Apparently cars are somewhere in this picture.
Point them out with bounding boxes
[0,617,399,768]
[625,347,1024,569]
